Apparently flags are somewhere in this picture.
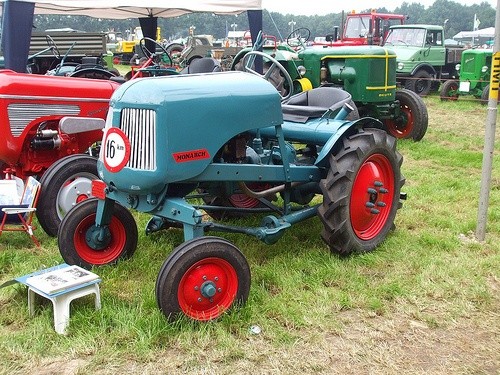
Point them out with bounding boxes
[475,16,480,28]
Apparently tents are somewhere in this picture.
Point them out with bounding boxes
[453,27,495,43]
[0,0,265,73]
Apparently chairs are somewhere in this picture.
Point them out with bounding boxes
[282,86,352,124]
[181,57,221,74]
[0,176,41,247]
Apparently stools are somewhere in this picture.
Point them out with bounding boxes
[27,283,101,335]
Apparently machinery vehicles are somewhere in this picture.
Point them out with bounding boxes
[0,9,499,209]
[55,48,409,332]
